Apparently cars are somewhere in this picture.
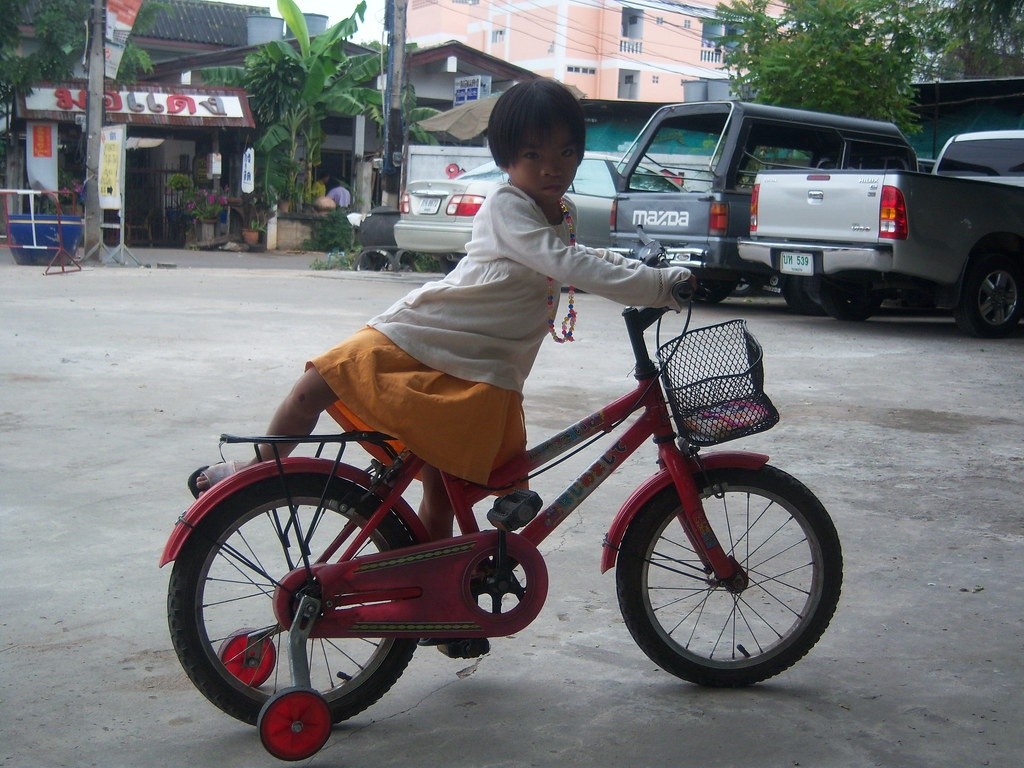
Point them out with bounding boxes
[393,152,690,277]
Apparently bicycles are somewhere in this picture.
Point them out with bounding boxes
[159,225,844,763]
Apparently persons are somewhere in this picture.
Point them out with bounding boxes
[312,171,330,204]
[326,176,351,213]
[188,78,699,646]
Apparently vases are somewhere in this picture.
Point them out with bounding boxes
[60,203,83,220]
[194,218,218,244]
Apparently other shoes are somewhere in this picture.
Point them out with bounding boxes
[187,458,238,502]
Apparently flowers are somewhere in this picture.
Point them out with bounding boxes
[58,170,84,205]
[185,184,231,223]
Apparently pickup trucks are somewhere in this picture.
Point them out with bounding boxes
[735,129,1024,339]
[609,98,920,313]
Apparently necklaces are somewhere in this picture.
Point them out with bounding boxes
[507,176,577,343]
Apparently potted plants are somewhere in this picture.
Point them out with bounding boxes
[241,220,266,246]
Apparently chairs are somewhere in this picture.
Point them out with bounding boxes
[126,207,153,247]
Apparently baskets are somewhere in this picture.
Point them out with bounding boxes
[654,317,780,447]
